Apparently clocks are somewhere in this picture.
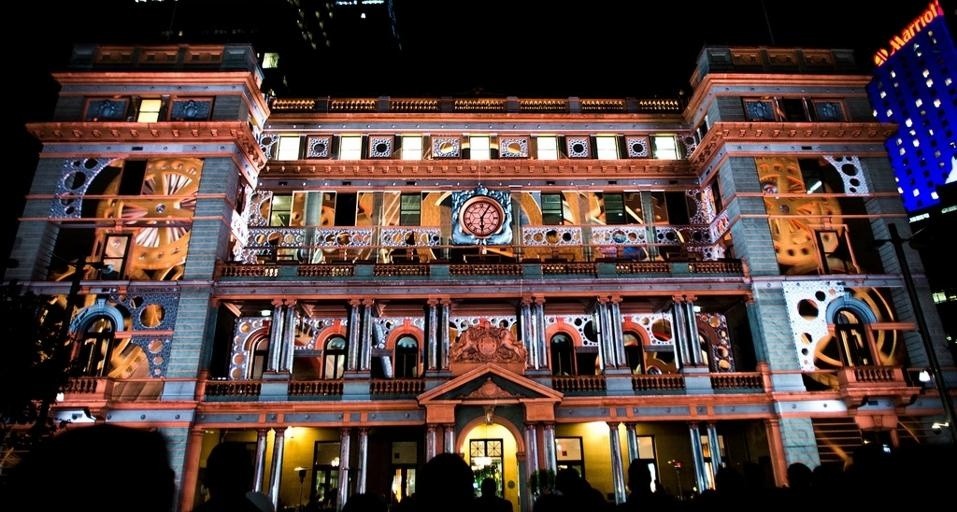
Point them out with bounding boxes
[459,193,504,238]
